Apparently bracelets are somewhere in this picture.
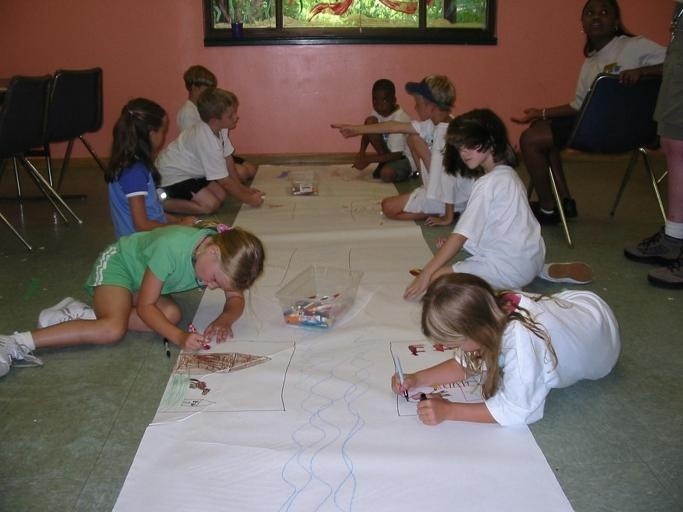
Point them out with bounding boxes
[542,108,546,119]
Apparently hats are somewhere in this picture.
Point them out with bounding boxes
[405,77,442,103]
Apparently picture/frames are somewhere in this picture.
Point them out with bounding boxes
[202,0,495,49]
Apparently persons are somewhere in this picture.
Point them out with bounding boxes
[350,78,417,182]
[151,87,264,217]
[174,63,256,184]
[508,0,670,224]
[404,107,597,303]
[102,97,207,242]
[1,220,266,374]
[328,73,482,228]
[622,0,683,289]
[392,273,623,426]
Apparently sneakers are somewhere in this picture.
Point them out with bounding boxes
[626,228,683,263]
[1,334,24,375]
[538,260,594,285]
[526,198,578,223]
[649,263,682,288]
[37,296,87,328]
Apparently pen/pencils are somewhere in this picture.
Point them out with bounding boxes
[394,354,409,402]
[163,338,170,358]
[284,293,348,329]
[188,322,211,350]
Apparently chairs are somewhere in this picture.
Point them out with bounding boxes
[524,64,668,250]
[0,66,111,254]
